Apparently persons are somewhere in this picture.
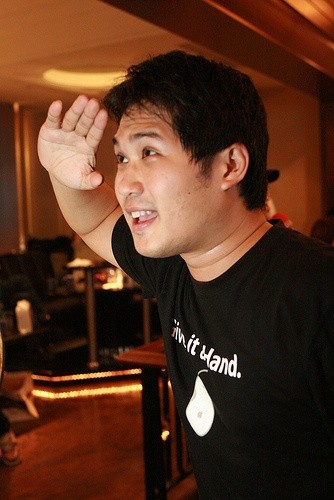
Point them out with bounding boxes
[36,51,334,500]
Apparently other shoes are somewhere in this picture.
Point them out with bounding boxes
[0,439,21,465]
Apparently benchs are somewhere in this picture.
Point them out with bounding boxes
[0,236,99,360]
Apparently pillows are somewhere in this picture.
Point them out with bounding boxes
[27,236,80,297]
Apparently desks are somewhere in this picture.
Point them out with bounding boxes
[117,340,199,500]
